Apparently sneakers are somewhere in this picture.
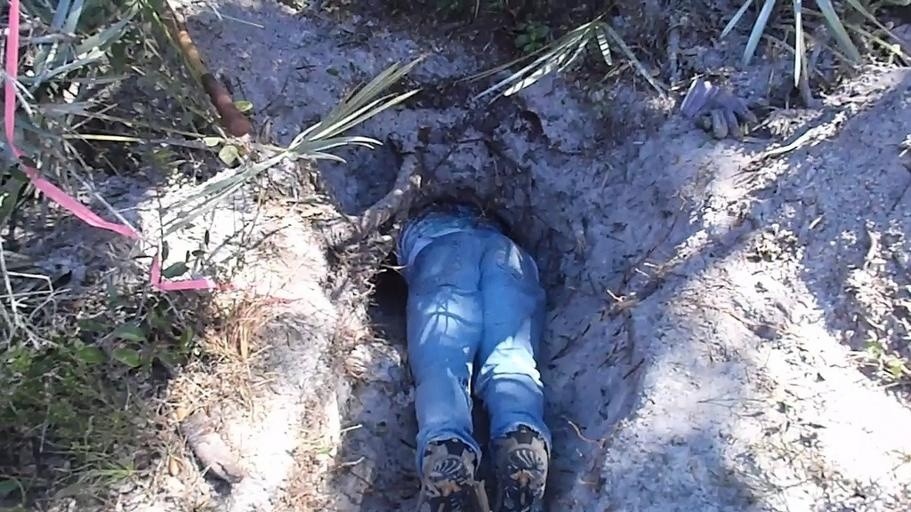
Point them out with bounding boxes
[415,437,483,512]
[487,423,550,512]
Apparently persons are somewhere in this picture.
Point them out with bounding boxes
[384,197,553,511]
